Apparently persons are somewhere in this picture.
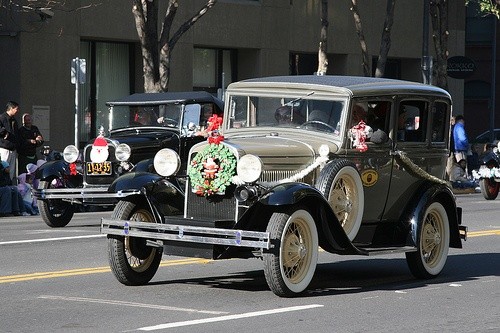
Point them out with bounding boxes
[351,104,380,142]
[157,116,210,127]
[446,117,456,182]
[454,116,473,180]
[274,107,297,127]
[18,113,44,175]
[0,155,48,216]
[451,159,481,189]
[0,101,19,180]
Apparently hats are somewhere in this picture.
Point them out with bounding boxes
[26,163,38,173]
[37,160,47,168]
[455,115,463,121]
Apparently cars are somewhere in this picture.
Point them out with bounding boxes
[31,91,224,228]
[96,75,468,299]
[471,129,500,158]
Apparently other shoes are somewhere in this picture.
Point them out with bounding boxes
[14,211,37,216]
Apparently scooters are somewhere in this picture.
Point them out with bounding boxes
[478,146,500,201]
